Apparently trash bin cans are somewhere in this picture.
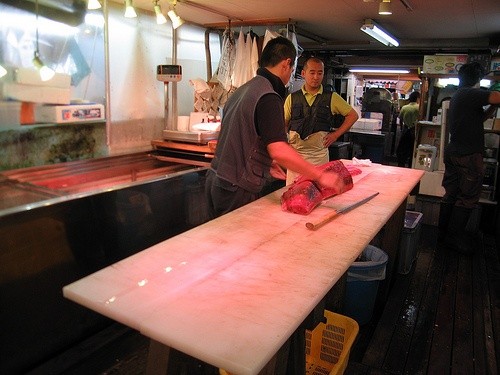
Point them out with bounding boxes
[325,245,389,334]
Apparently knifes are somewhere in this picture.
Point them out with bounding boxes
[307,192,379,230]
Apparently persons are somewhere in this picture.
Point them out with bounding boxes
[397,92,419,168]
[205,36,344,220]
[283,57,359,187]
[433,62,500,261]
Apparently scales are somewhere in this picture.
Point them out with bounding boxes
[156,64,221,146]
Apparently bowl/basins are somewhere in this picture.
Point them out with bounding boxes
[208,142,217,151]
[483,148,497,157]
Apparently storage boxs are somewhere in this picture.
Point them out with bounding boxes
[399,208,425,274]
[305,310,359,375]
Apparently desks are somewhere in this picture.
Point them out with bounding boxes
[63,159,426,375]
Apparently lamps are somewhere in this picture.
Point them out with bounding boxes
[378,0,392,14]
[360,19,399,47]
[154,0,168,25]
[169,0,184,30]
[31,0,55,81]
[124,1,137,17]
